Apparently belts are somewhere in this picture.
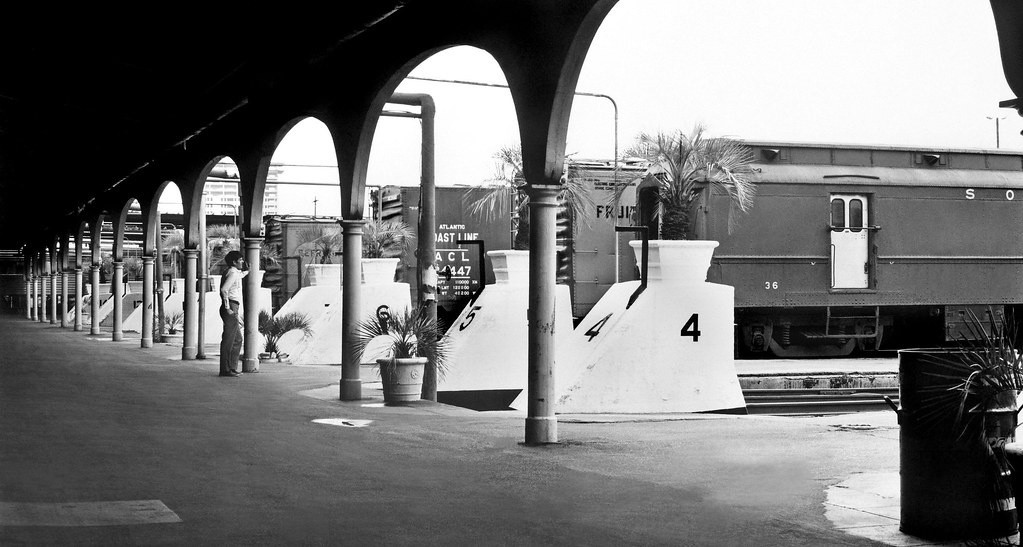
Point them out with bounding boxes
[230,300,239,305]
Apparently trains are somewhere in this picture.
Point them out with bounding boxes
[507,135,1023,363]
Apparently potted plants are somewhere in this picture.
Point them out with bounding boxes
[152,311,183,344]
[362,217,417,283]
[84,228,185,296]
[346,296,451,403]
[289,219,342,286]
[82,297,92,324]
[601,122,760,285]
[205,224,286,291]
[444,137,599,285]
[257,311,314,364]
[908,306,1023,547]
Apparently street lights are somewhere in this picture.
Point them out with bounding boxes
[986,115,1008,149]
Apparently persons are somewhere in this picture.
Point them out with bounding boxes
[218,250,250,377]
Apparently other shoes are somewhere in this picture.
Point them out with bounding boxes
[219,371,234,377]
[231,369,241,374]
